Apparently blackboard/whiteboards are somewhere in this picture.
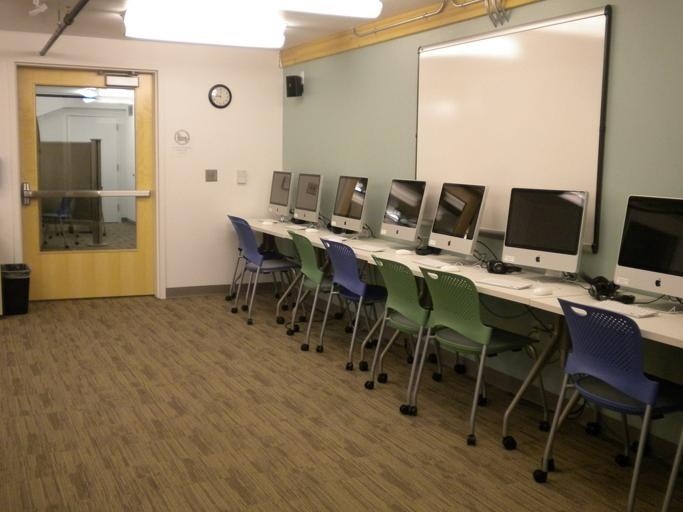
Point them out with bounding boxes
[415,5,612,254]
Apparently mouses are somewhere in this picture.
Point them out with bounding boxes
[534,286,553,296]
[441,265,460,272]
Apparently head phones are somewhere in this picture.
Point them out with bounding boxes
[486,260,522,274]
[588,275,617,300]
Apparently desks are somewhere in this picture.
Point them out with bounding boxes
[247,219,683,449]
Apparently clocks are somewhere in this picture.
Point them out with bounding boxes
[209,84,232,108]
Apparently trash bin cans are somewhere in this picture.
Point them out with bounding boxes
[1,263,32,315]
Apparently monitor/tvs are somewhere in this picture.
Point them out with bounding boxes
[259,171,488,267]
[502,188,588,284]
[614,195,683,299]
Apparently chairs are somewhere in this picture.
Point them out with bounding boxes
[364,255,482,414]
[287,230,372,350]
[409,266,550,445]
[662,434,683,512]
[316,238,413,371]
[68,196,106,236]
[225,215,291,301]
[533,298,683,512]
[42,198,79,248]
[232,221,306,324]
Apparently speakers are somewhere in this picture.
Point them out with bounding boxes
[287,76,302,97]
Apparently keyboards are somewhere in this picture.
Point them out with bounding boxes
[476,276,533,290]
[592,299,656,317]
